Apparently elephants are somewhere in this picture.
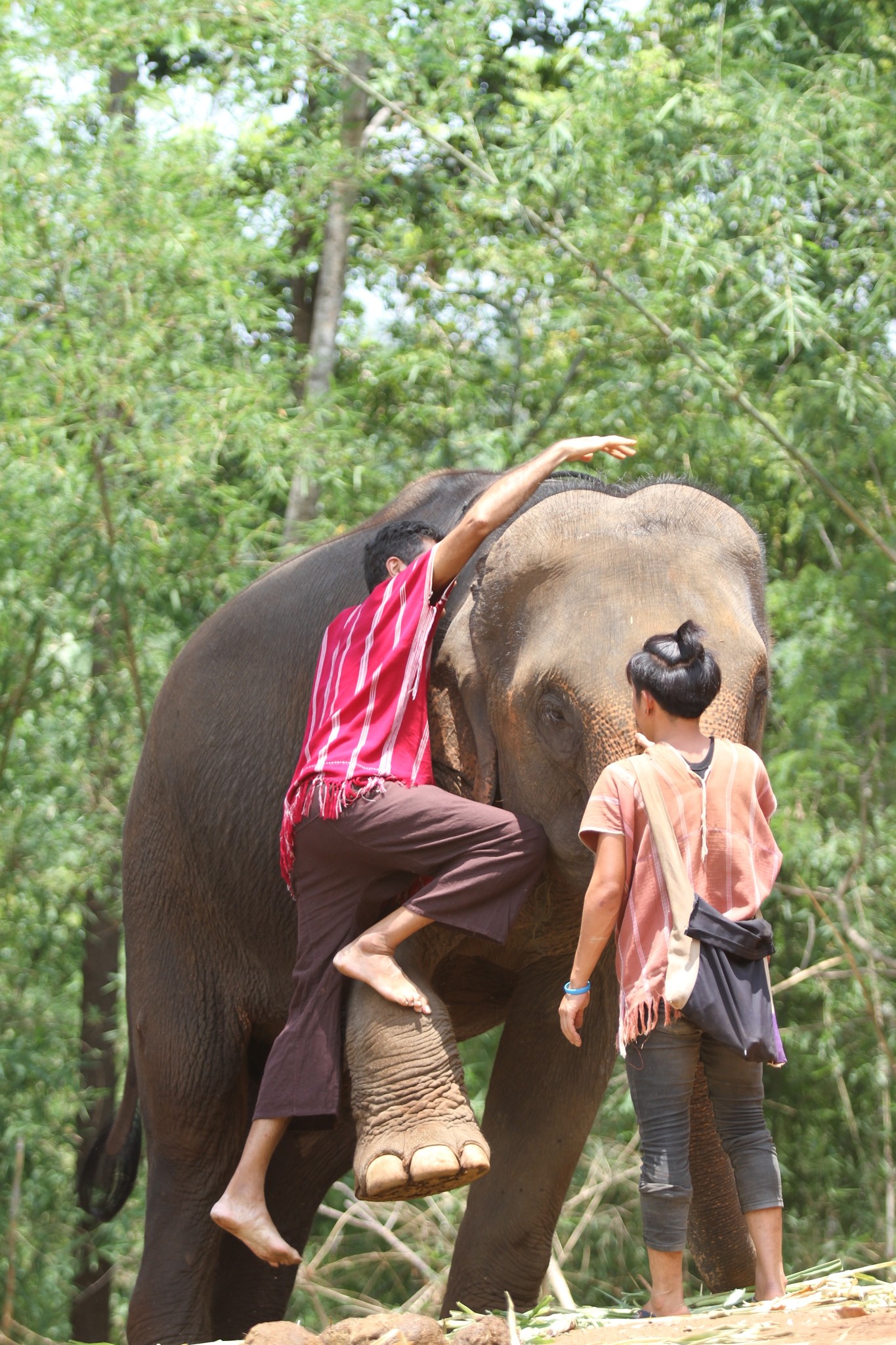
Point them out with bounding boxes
[86,471,770,1344]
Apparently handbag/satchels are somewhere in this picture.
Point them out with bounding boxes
[664,894,786,1068]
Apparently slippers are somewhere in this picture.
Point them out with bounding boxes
[636,1309,657,1319]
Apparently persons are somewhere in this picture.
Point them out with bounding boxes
[206,435,635,1269]
[559,619,786,1320]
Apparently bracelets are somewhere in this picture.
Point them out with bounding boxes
[564,981,590,994]
